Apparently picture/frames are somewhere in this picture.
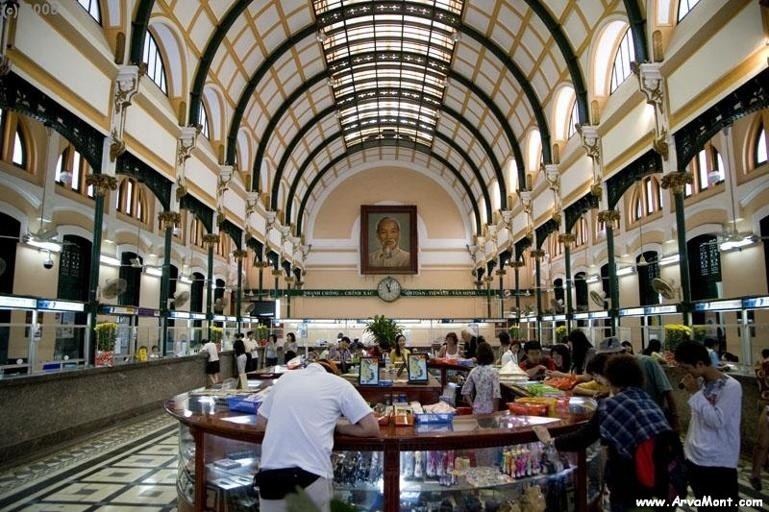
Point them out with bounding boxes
[359,357,379,385]
[408,353,428,382]
[361,205,417,274]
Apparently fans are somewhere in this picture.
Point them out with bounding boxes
[590,291,612,310]
[651,277,682,300]
[551,299,567,312]
[245,304,255,314]
[167,291,189,310]
[212,297,227,310]
[96,278,127,301]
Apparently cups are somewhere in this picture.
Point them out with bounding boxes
[468,467,502,486]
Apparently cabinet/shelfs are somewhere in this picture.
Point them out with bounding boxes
[165,364,605,511]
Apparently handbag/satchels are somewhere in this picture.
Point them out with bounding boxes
[546,377,576,389]
[257,470,294,500]
[634,435,670,489]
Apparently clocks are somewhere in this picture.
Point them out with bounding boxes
[377,277,401,302]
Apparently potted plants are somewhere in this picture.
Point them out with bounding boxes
[664,324,691,366]
[258,326,268,346]
[509,326,519,343]
[363,315,404,360]
[210,326,222,352]
[96,323,116,366]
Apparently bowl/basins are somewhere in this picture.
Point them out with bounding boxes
[506,398,551,415]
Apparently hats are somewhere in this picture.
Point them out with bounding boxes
[315,358,338,376]
[594,336,626,355]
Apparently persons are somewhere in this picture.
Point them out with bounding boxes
[368,216,411,267]
[137,327,768,511]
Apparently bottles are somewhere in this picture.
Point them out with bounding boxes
[404,450,457,487]
[544,392,570,416]
[384,393,407,405]
[501,444,542,478]
